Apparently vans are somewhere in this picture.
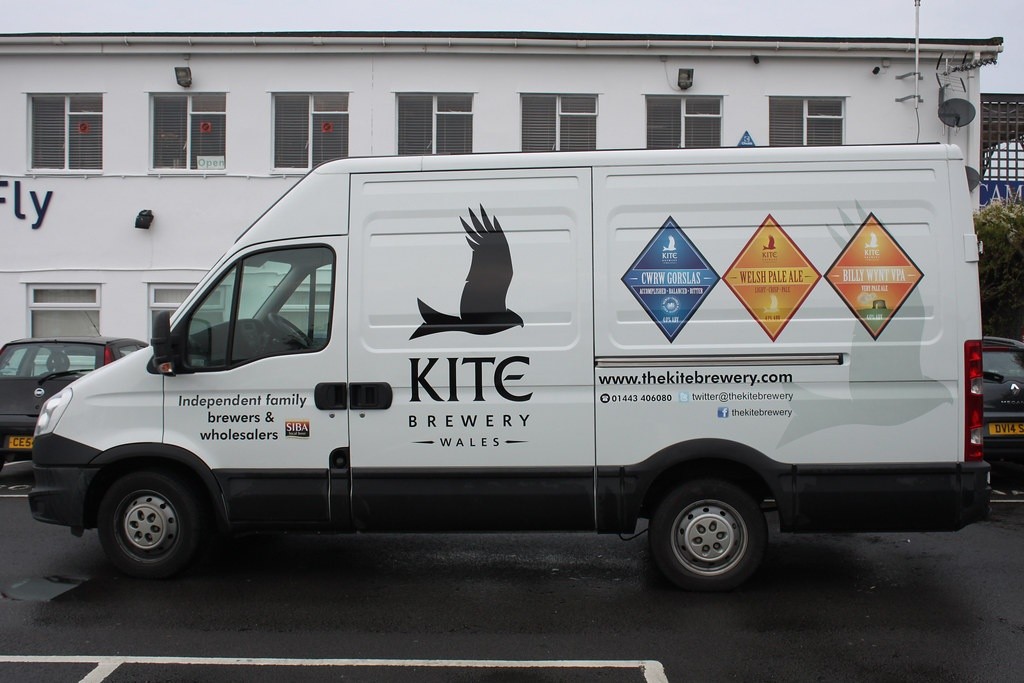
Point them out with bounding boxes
[23,137,993,598]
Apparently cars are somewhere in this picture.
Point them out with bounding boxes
[981,333,1024,495]
[0,335,150,483]
